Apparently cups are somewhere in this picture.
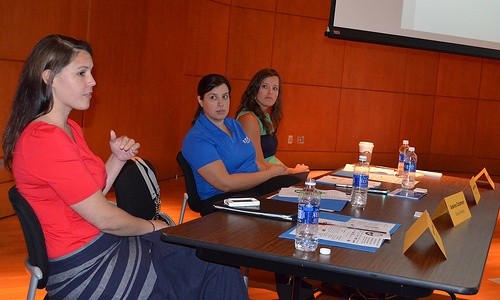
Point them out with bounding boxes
[359,142,374,166]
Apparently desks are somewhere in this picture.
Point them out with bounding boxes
[161,165,500,300]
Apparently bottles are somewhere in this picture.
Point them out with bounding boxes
[402,147,417,189]
[295,179,320,252]
[351,156,370,208]
[398,140,410,175]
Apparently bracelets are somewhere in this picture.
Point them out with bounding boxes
[147,220,155,232]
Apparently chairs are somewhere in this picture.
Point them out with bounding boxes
[8,185,50,300]
[176,150,201,225]
[113,156,176,227]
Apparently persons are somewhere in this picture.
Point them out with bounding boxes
[182,73,315,300]
[235,68,313,181]
[2,34,250,300]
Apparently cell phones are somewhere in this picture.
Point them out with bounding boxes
[225,198,260,206]
[368,188,387,194]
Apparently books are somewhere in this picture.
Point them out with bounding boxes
[214,196,299,221]
